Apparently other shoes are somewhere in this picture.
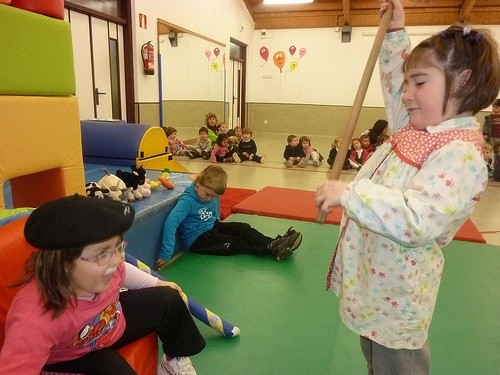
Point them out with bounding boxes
[313,160,318,167]
[185,151,193,159]
[223,158,234,162]
[233,153,241,163]
[201,151,209,160]
[284,161,293,168]
[260,155,266,164]
[296,161,306,168]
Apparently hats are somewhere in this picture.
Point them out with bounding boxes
[24,193,136,248]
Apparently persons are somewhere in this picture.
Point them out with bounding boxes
[156,165,303,269]
[481,98,500,184]
[161,112,323,167]
[313,0,500,375]
[327,120,392,171]
[0,192,205,375]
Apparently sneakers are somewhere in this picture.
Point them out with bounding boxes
[274,232,302,262]
[275,226,296,240]
[158,353,197,375]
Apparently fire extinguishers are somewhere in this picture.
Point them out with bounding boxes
[141,40,154,75]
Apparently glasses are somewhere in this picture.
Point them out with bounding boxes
[77,240,128,266]
